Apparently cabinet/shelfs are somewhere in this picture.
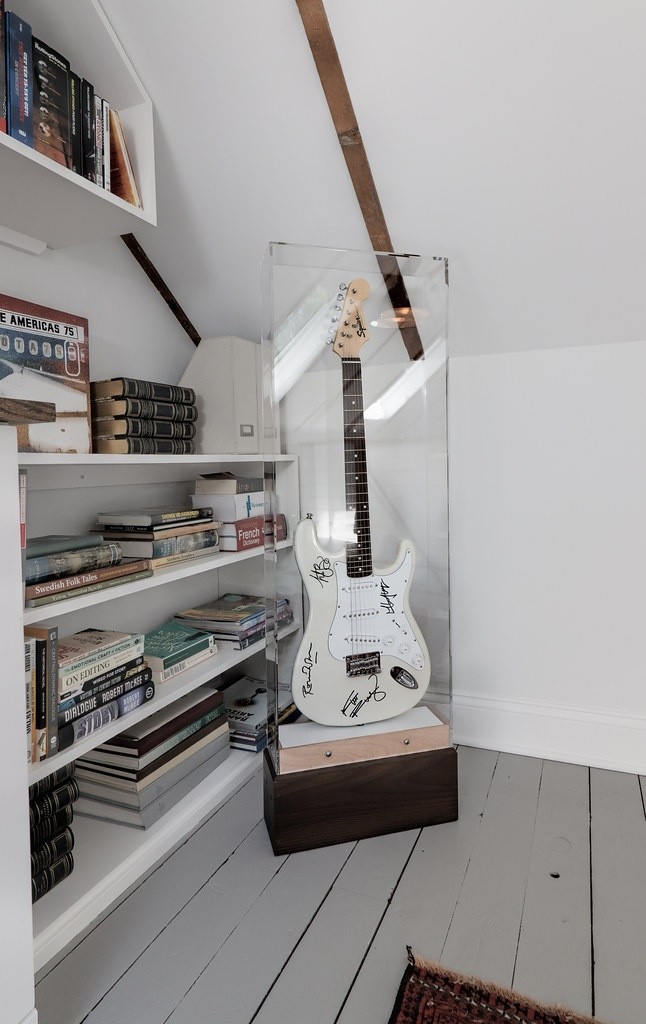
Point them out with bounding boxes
[20,454,304,976]
[0,0,159,253]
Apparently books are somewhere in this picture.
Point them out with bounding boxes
[220,674,303,754]
[29,760,80,906]
[89,504,223,570]
[172,593,295,651]
[189,471,287,553]
[19,469,154,609]
[0,0,143,209]
[143,620,218,684]
[91,376,198,454]
[24,623,156,763]
[72,686,230,831]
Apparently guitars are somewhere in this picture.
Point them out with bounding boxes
[290,273,433,730]
